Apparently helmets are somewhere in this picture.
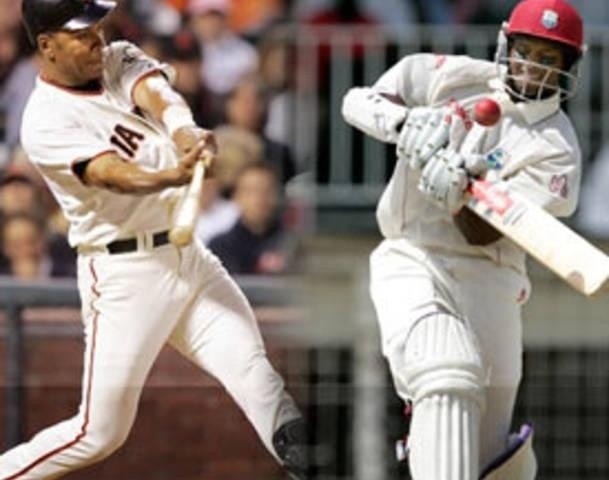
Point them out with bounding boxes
[494,0,583,105]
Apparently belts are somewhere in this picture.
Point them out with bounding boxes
[107,232,167,254]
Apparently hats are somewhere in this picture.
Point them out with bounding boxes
[22,0,118,31]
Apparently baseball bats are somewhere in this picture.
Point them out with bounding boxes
[167,158,206,246]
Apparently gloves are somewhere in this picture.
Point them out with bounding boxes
[396,105,453,169]
[417,147,488,216]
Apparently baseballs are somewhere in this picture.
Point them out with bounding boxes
[473,98,499,125]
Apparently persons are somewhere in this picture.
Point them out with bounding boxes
[0,0,336,480]
[341,0,587,480]
[1,0,303,278]
